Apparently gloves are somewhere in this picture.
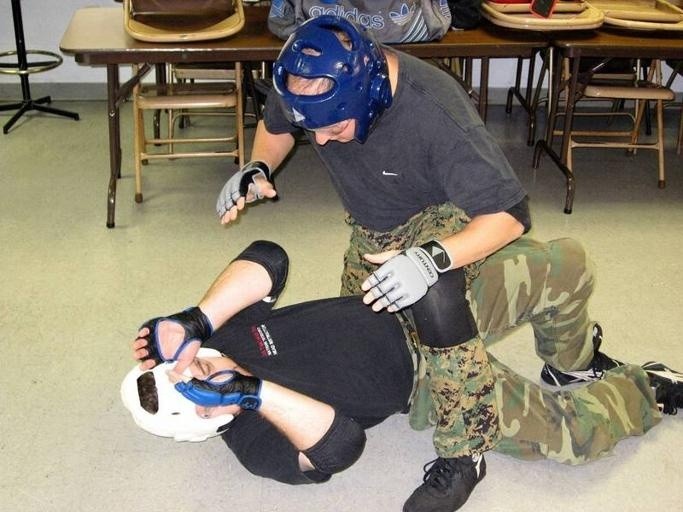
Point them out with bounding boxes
[174,371,262,411]
[134,306,212,367]
[369,238,452,312]
[215,160,272,218]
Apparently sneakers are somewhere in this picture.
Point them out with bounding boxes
[538,326,624,389]
[404,455,485,511]
[639,360,682,414]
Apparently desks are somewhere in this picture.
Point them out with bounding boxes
[60,5,548,230]
[532,32,680,214]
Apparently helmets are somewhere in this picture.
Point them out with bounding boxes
[122,363,234,442]
[272,14,392,143]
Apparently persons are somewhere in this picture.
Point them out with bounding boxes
[214,13,532,512]
[118,237,682,486]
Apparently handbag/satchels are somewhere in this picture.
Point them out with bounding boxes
[268,1,451,44]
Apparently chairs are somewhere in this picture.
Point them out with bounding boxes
[128,60,246,209]
[562,57,669,199]
